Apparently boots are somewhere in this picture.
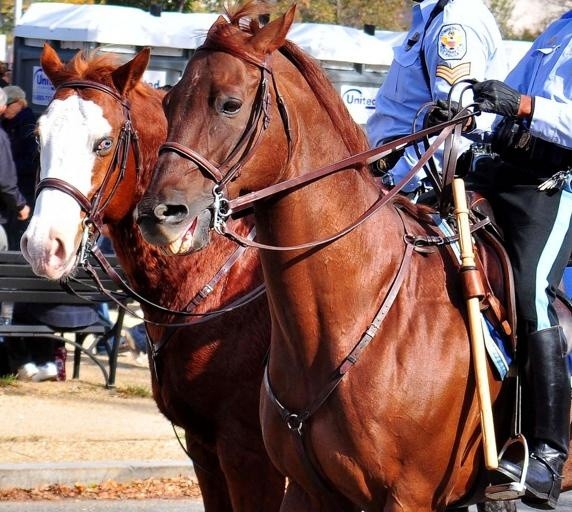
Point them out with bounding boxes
[491,325,571,511]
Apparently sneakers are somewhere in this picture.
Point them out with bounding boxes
[18,362,58,381]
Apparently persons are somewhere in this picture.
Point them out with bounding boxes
[403,1,572,511]
[361,2,509,208]
[1,60,116,385]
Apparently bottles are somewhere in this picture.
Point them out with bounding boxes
[54,342,68,382]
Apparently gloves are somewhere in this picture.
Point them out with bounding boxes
[426,98,469,139]
[471,79,520,118]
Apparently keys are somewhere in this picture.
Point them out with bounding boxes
[536,170,568,193]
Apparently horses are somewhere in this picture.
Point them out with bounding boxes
[132,0,572,512]
[20,43,272,512]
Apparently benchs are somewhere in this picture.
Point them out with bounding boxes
[1,247,143,387]
